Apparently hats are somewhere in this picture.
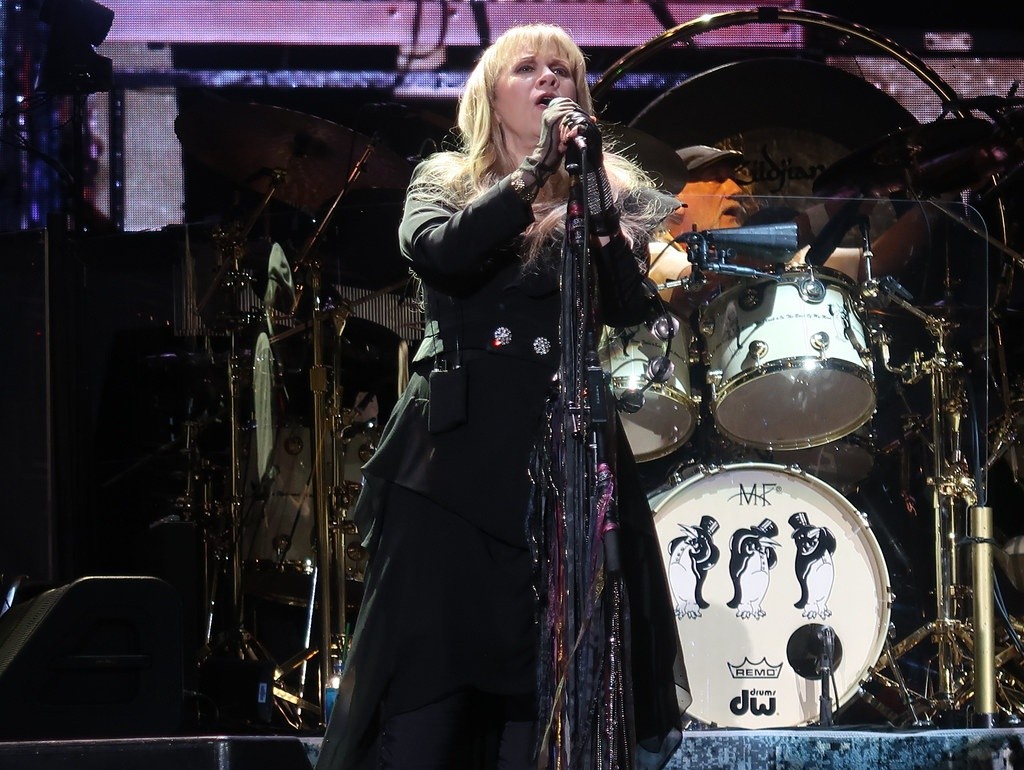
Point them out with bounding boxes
[675,144,744,175]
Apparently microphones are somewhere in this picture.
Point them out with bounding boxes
[549,96,587,150]
[805,189,869,267]
[818,625,836,643]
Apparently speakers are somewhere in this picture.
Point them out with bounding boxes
[0,576,188,741]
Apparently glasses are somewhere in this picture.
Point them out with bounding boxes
[686,160,755,185]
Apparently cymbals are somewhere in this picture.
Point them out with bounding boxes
[174,99,415,215]
[812,117,997,211]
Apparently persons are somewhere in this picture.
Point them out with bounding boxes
[647,143,1018,259]
[316,20,688,769]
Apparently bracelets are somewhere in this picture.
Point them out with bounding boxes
[570,165,613,216]
[508,172,536,204]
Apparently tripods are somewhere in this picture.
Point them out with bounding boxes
[837,260,1024,731]
[191,144,370,735]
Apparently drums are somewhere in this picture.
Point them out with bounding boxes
[697,264,886,456]
[597,301,700,465]
[643,457,895,734]
[233,411,385,615]
